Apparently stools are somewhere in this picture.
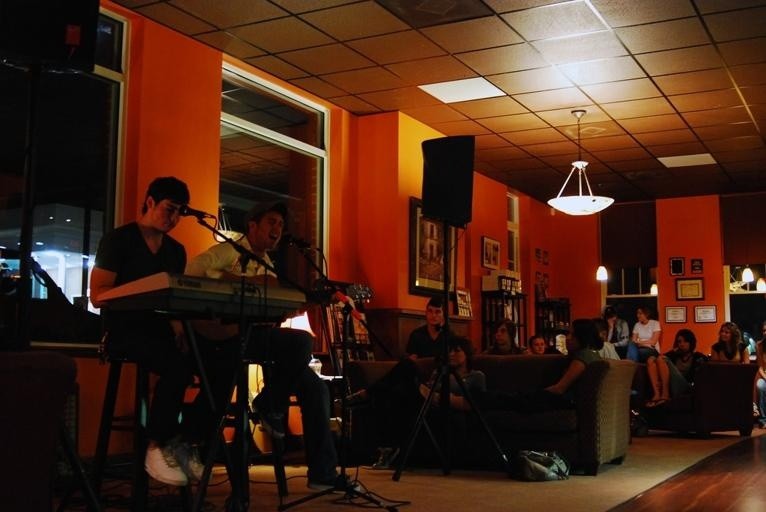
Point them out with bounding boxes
[196,350,296,503]
[87,345,198,511]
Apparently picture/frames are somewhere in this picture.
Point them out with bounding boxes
[665,305,688,323]
[403,193,460,303]
[674,277,705,301]
[693,304,718,324]
[480,234,502,271]
[668,256,685,277]
[453,285,475,320]
[690,258,705,274]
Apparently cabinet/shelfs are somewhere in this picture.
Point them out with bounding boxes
[478,289,528,355]
[534,295,571,343]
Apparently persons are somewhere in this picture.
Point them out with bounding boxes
[184,199,360,495]
[335,336,488,471]
[90,176,212,487]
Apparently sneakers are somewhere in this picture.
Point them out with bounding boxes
[176,442,207,480]
[307,474,341,491]
[144,446,190,486]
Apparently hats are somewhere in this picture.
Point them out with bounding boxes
[244,200,288,227]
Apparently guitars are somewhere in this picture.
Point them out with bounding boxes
[197,271,371,342]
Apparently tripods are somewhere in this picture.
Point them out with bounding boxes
[274,253,400,512]
[380,230,514,484]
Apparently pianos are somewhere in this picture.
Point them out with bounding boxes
[95,272,307,317]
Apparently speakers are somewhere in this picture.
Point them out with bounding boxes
[421,134,476,229]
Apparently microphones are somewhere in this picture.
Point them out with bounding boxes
[282,224,315,252]
[176,203,212,218]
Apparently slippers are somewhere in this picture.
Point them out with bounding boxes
[644,397,671,407]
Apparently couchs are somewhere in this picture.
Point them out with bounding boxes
[629,350,762,445]
[336,345,638,483]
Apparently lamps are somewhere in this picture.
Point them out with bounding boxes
[546,108,617,220]
[740,225,755,283]
[593,180,610,281]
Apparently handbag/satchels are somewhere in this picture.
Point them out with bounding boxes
[517,447,570,480]
